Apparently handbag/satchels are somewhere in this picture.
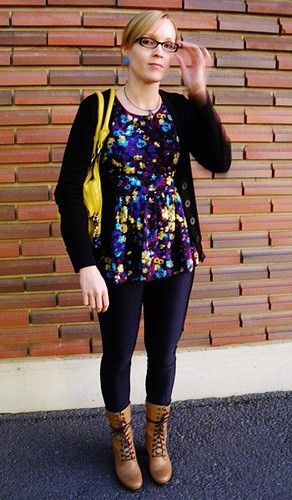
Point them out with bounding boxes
[57,87,115,241]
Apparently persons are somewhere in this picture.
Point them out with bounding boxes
[52,9,233,491]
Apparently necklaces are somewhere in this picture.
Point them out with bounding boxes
[123,81,161,118]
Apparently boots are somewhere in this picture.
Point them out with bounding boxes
[105,402,143,492]
[144,400,173,485]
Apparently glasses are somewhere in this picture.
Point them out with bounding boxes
[134,36,179,52]
